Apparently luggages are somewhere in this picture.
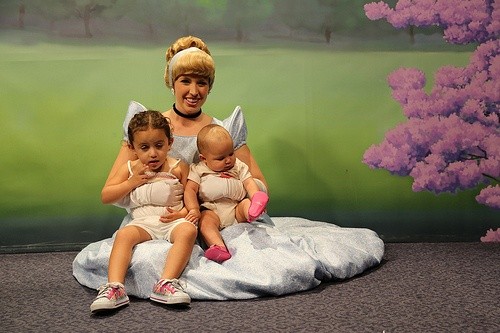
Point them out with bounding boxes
[205,245,230,262]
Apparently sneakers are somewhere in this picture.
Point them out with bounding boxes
[150,278,191,304]
[90,282,129,313]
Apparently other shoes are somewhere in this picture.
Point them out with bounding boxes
[247,191,269,223]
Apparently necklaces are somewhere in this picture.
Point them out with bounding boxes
[173,103,202,119]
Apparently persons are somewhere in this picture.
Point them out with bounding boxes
[90,35,270,314]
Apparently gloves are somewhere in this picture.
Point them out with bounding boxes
[200,175,267,201]
[113,179,183,209]
